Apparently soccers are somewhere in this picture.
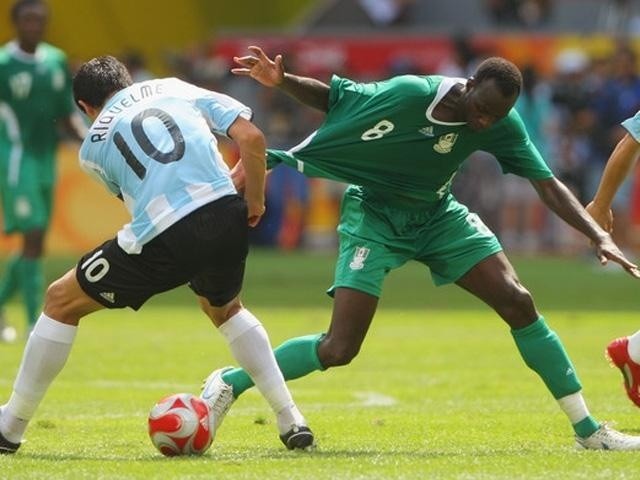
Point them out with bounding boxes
[149,393,213,457]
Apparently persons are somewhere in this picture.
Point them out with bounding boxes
[585,108,640,409]
[0,0,94,337]
[198,45,639,452]
[0,56,315,456]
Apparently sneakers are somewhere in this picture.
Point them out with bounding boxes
[199,367,238,431]
[0,435,23,455]
[605,338,640,406]
[574,422,640,452]
[276,405,313,450]
